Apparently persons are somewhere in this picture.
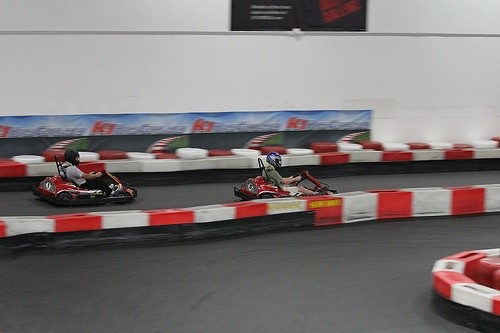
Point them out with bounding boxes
[57,149,122,196]
[262,150,321,195]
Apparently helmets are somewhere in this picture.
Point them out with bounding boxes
[266,151,282,169]
[64,149,80,166]
